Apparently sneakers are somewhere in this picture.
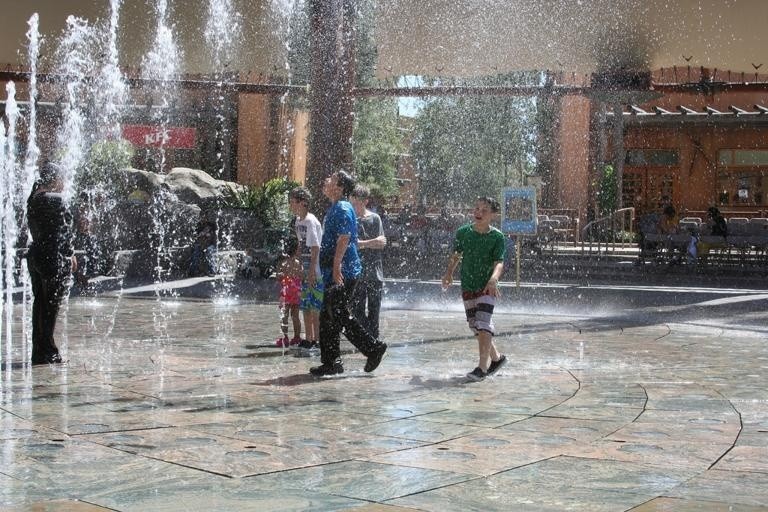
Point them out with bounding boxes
[278,334,316,351]
[32,352,62,365]
[486,355,507,374]
[311,362,344,376]
[364,340,386,372]
[467,367,487,381]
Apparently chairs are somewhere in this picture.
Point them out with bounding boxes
[383,213,581,279]
[636,215,768,279]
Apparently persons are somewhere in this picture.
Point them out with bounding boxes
[440,195,509,382]
[72,192,545,292]
[275,235,305,348]
[288,185,323,352]
[309,171,388,377]
[349,185,388,342]
[658,207,684,266]
[24,163,77,367]
[692,207,727,266]
